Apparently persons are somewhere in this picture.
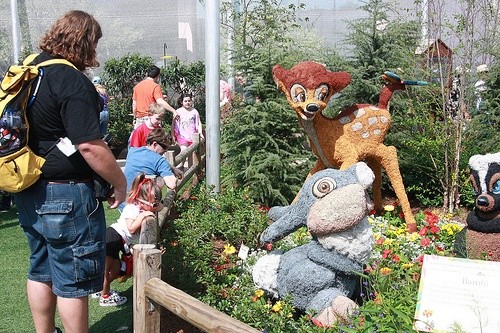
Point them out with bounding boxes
[129,102,166,148]
[132,65,180,127]
[170,93,205,170]
[11,9,127,333]
[117,128,184,282]
[93,84,111,139]
[91,173,164,307]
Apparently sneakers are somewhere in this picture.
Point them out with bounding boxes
[99,293,127,306]
[92,290,117,299]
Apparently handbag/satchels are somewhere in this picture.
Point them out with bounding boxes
[116,254,133,283]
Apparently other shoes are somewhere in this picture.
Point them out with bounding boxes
[54,327,63,333]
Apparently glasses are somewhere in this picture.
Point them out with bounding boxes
[158,142,168,150]
[135,197,164,208]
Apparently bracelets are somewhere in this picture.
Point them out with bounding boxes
[133,118,136,120]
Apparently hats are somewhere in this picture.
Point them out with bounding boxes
[92,77,101,83]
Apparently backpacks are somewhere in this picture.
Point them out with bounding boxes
[0,54,83,193]
